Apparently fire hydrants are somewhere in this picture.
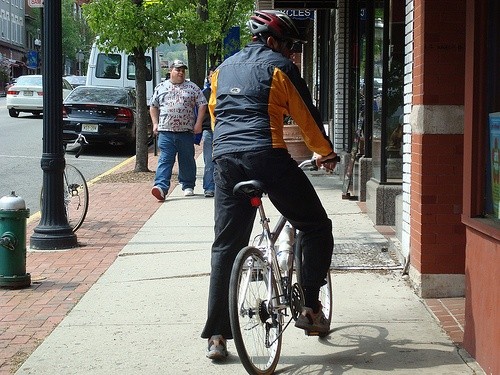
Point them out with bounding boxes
[0,190,33,290]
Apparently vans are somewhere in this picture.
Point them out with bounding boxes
[85,35,161,109]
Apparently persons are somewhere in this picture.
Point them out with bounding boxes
[149,60,205,200]
[200,67,215,196]
[200,12,339,362]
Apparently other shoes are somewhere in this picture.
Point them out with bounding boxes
[204,190,214,197]
[151,186,165,201]
[184,188,194,195]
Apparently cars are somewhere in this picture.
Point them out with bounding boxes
[63,86,158,156]
[6,74,75,118]
[360,78,384,92]
[61,76,88,86]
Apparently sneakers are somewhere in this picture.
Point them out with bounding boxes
[295,301,330,331]
[206,335,228,359]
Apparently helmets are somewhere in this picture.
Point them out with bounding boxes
[249,10,301,42]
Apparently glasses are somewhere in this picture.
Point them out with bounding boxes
[285,40,295,50]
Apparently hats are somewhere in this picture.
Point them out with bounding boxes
[170,60,187,70]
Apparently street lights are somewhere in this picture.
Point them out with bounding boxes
[35,38,42,78]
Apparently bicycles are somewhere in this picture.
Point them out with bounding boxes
[228,156,340,375]
[40,163,90,234]
